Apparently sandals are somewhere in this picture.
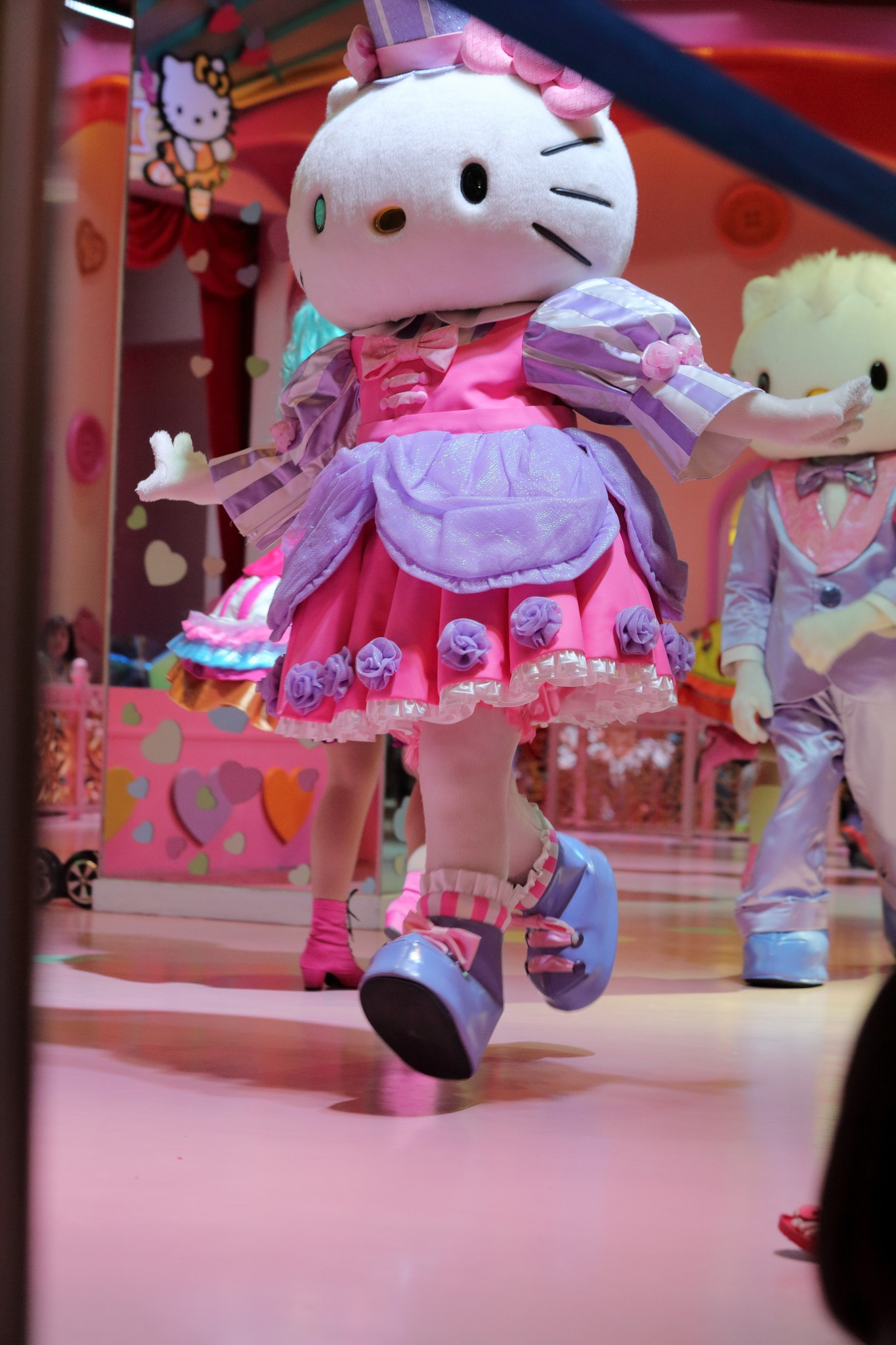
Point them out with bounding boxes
[778,1205,820,1252]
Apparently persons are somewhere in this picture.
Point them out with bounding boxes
[168,304,427,988]
[39,616,77,683]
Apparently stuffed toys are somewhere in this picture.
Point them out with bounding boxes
[719,251,896,984]
[138,1,871,1079]
